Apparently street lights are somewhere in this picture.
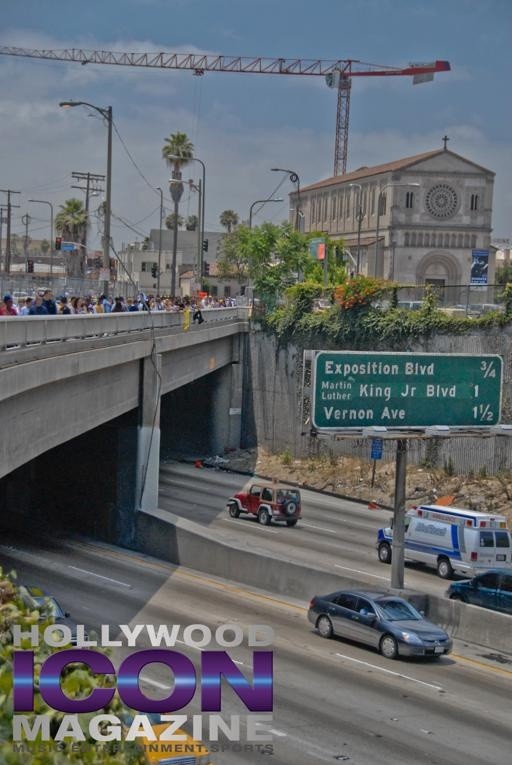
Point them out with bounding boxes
[59,101,112,294]
[156,155,205,294]
[249,169,304,231]
[343,182,421,279]
[28,199,53,272]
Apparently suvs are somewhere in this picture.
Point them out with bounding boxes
[226,483,302,526]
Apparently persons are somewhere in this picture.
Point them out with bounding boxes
[0,286,246,318]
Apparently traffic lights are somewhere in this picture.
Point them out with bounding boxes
[151,262,157,279]
[28,260,33,272]
[56,238,61,250]
[204,261,210,277]
[203,238,208,252]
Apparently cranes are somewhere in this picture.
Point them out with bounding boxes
[0,46,451,177]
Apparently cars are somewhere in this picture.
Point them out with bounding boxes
[445,569,512,615]
[308,590,453,659]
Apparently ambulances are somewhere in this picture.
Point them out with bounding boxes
[376,505,512,579]
